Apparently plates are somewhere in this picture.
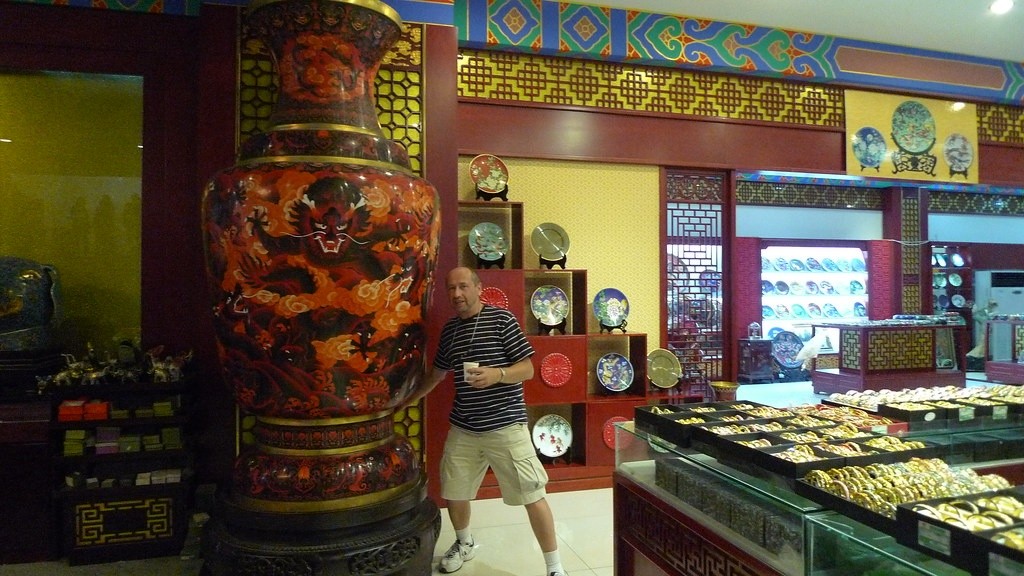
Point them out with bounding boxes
[932,273,963,287]
[468,222,509,261]
[892,100,935,152]
[532,414,572,458]
[761,256,865,271]
[931,253,964,267]
[943,132,973,170]
[647,348,681,388]
[761,280,865,295]
[932,294,966,308]
[851,127,887,167]
[469,153,510,193]
[592,288,629,327]
[530,222,571,261]
[596,352,634,392]
[530,285,570,326]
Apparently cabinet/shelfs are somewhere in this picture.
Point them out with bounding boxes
[0,369,63,563]
[57,374,194,567]
[738,339,774,384]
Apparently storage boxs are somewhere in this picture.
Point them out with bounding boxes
[1,396,186,455]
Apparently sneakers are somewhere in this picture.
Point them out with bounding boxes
[439,534,475,572]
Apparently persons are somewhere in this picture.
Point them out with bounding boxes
[394,266,565,576]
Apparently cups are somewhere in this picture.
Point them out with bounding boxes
[463,362,478,382]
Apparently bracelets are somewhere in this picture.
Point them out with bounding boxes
[498,367,505,383]
[650,382,1024,554]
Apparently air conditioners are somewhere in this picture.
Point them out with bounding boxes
[975,269,1024,363]
